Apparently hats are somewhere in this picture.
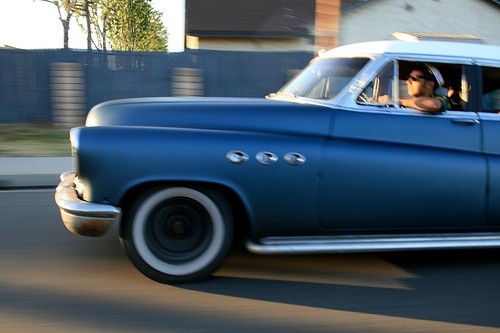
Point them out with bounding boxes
[417,62,445,88]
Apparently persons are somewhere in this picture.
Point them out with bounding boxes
[483,76,500,113]
[363,61,454,113]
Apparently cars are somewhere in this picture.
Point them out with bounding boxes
[55,40,500,286]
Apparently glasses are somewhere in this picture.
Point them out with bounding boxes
[408,74,426,81]
